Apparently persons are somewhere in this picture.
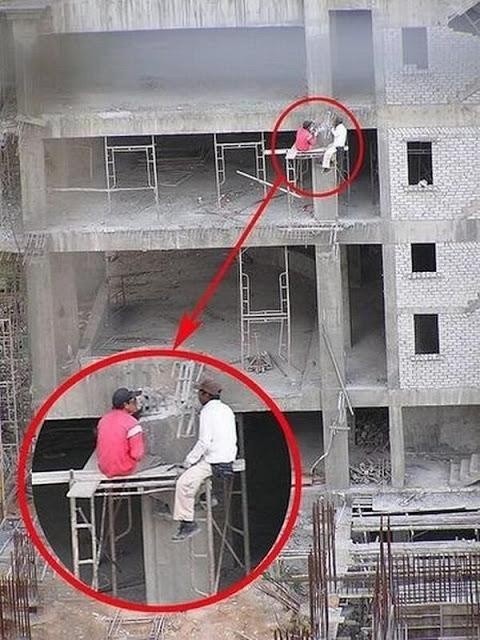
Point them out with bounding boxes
[321,117,347,172]
[96,388,173,523]
[170,378,237,542]
[296,121,322,166]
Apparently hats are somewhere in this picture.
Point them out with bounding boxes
[112,387,142,407]
[193,379,223,396]
[303,120,312,129]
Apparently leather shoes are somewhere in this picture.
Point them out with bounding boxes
[321,169,332,176]
[201,493,219,509]
[171,522,201,543]
